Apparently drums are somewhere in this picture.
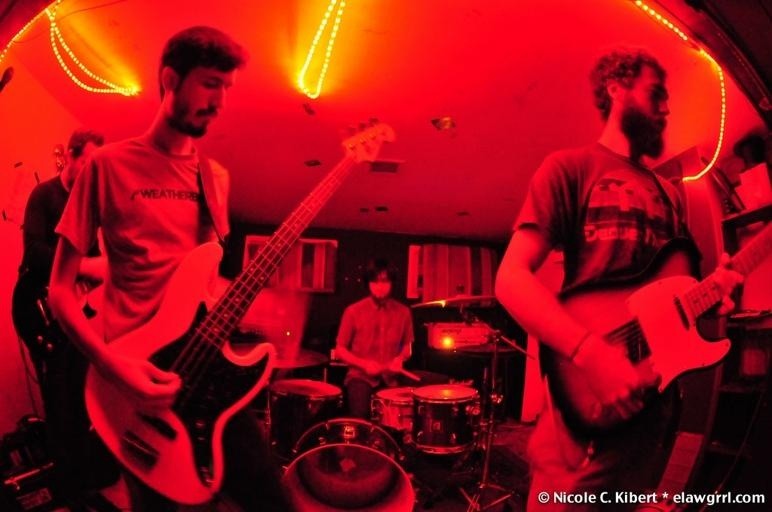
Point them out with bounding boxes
[369,385,413,436]
[411,386,480,456]
[266,379,345,454]
[279,417,419,512]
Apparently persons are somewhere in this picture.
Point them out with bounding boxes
[11,123,106,432]
[492,46,748,512]
[46,20,305,512]
[332,256,416,421]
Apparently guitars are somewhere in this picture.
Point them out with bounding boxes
[86,117,396,506]
[538,221,772,441]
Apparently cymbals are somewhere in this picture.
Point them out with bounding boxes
[410,293,496,309]
[456,343,513,351]
